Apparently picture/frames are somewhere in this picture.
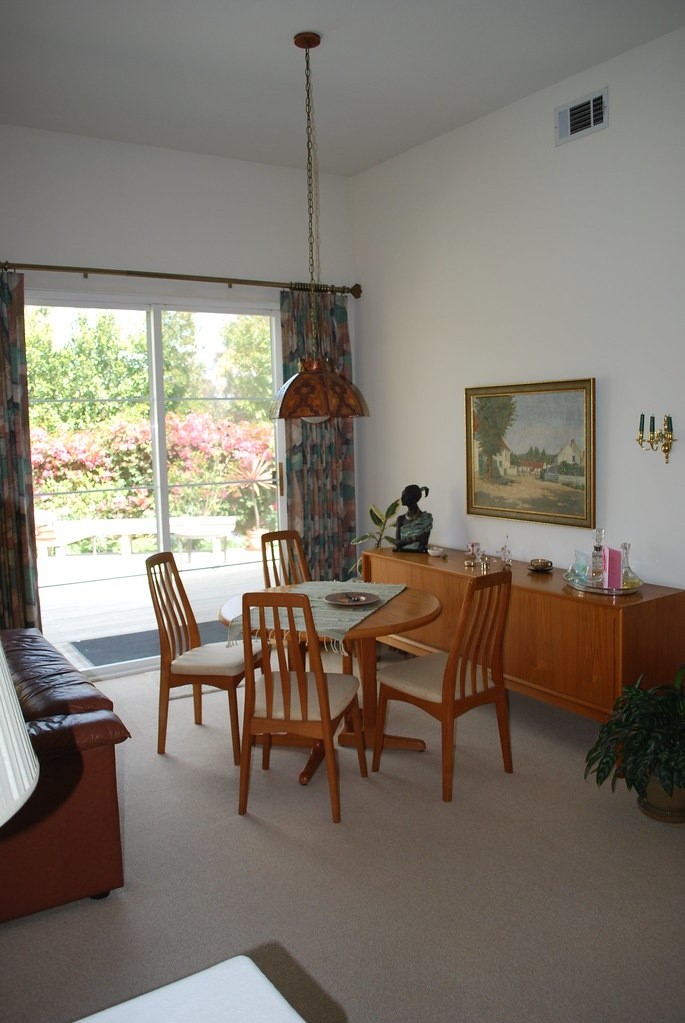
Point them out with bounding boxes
[464,376,596,530]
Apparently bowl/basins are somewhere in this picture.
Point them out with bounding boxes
[428,547,444,557]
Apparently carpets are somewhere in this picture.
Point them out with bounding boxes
[70,620,262,670]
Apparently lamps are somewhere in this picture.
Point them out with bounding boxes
[269,32,364,424]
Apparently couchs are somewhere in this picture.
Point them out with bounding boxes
[1,627,132,924]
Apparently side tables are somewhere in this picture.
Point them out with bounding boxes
[362,543,685,779]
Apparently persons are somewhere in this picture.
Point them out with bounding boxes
[392,484,434,553]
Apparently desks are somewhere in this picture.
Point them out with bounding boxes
[217,579,443,786]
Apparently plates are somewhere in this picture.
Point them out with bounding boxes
[527,565,553,572]
[324,591,379,606]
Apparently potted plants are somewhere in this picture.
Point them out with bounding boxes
[582,663,685,824]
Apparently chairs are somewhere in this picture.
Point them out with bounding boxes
[146,551,272,767]
[238,592,369,823]
[261,530,361,675]
[371,570,514,802]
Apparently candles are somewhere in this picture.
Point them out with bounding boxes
[636,411,678,464]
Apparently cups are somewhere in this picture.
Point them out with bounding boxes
[592,545,603,578]
[531,559,553,569]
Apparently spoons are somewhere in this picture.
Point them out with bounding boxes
[345,593,359,602]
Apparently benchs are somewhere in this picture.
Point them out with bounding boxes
[35,515,239,573]
